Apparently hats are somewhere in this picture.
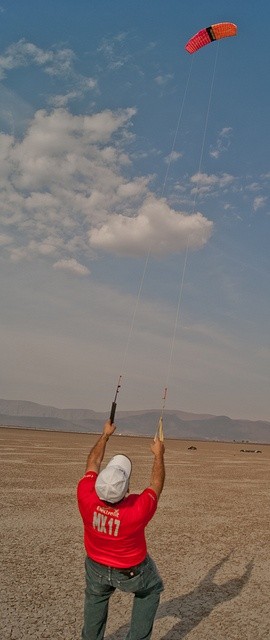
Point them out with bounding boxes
[95,454,132,504]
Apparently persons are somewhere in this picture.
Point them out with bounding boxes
[75,411,168,639]
